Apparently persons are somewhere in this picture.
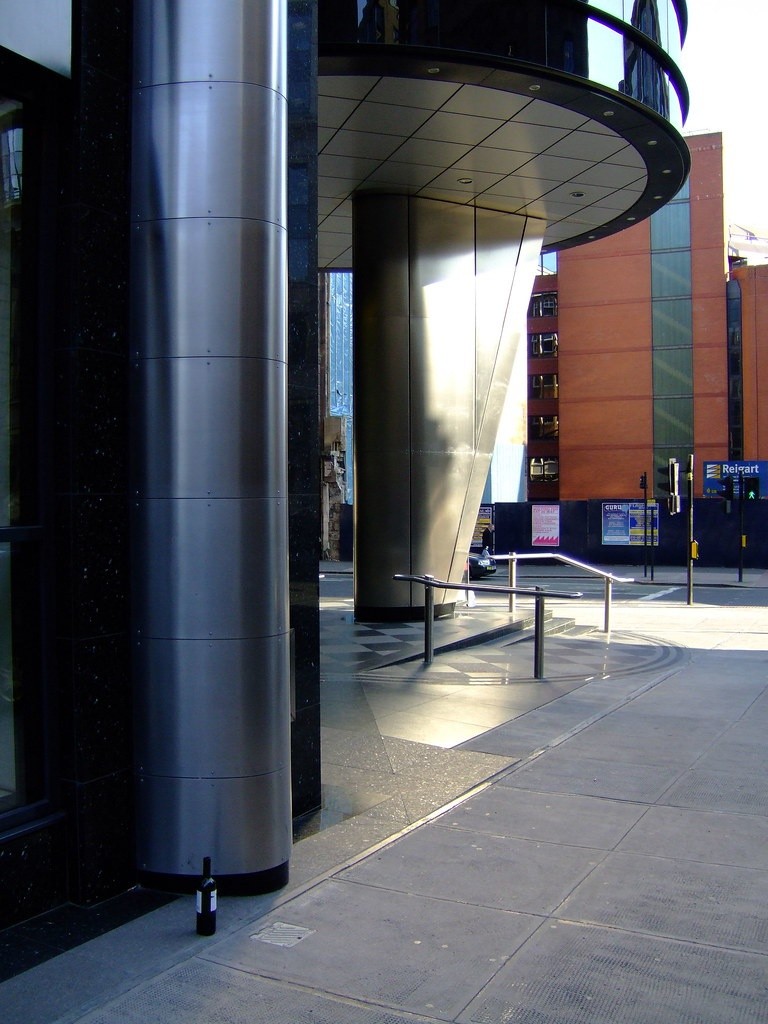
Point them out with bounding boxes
[482,524,495,556]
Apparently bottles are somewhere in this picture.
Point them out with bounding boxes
[196,857,217,937]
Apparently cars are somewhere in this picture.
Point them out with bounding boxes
[462,551,497,580]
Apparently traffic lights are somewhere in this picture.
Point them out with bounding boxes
[656,457,677,492]
[639,475,648,489]
[690,540,700,559]
[741,534,748,548]
[744,476,760,504]
[654,494,681,514]
[715,474,734,501]
